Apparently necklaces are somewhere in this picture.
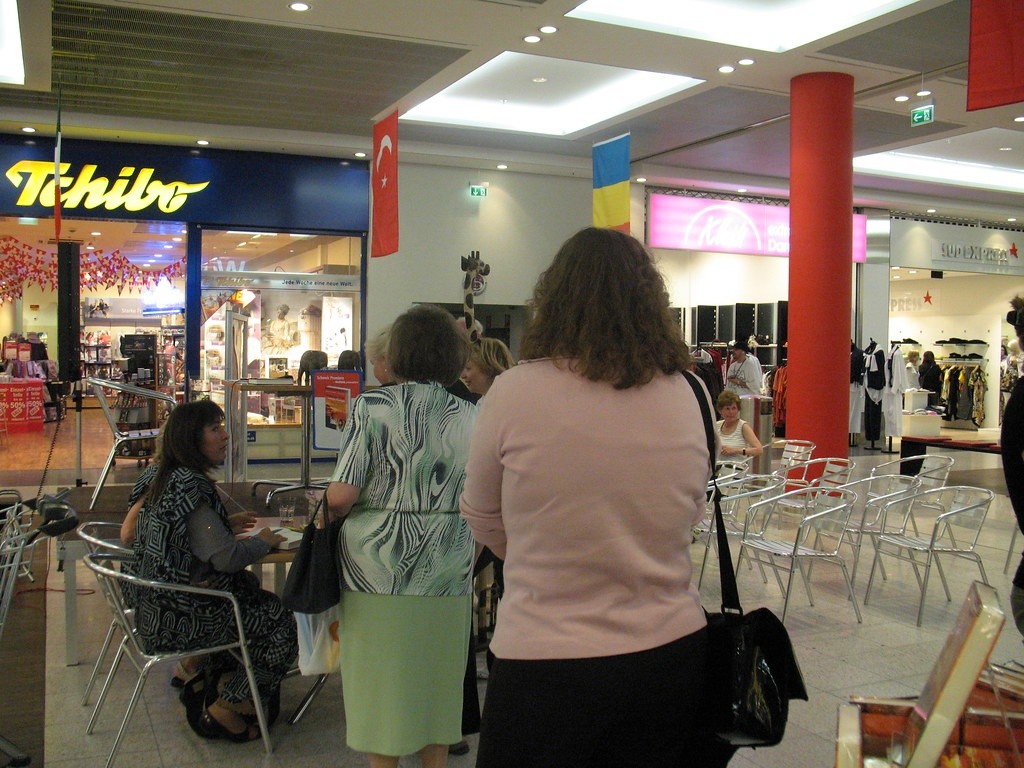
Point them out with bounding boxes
[732,355,748,380]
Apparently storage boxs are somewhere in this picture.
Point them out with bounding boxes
[268,398,282,422]
[111,357,131,373]
[144,369,150,378]
[162,336,175,351]
[281,409,287,420]
[137,368,144,378]
[287,409,295,423]
[131,374,137,380]
[174,336,185,350]
[247,395,261,414]
[268,358,288,380]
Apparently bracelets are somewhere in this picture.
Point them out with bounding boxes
[737,380,740,385]
[742,447,746,457]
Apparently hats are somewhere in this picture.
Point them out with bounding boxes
[734,341,751,354]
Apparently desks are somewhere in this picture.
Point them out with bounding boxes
[220,378,380,508]
[235,516,309,601]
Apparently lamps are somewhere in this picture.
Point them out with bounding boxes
[143,307,185,317]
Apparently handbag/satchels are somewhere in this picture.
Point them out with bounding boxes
[294,602,342,677]
[705,607,810,750]
[180,666,281,735]
[285,485,345,613]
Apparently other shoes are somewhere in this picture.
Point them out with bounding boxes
[171,663,226,691]
[200,708,265,742]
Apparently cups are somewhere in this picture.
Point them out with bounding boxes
[278,496,295,530]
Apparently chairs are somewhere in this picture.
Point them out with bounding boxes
[75,520,135,706]
[732,484,863,625]
[862,486,996,627]
[848,454,956,582]
[87,377,178,512]
[695,460,752,592]
[806,473,924,605]
[740,438,816,532]
[0,393,11,454]
[80,551,273,768]
[772,457,853,582]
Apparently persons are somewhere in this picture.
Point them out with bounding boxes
[118,395,300,742]
[999,292,1024,641]
[905,350,942,407]
[315,302,519,768]
[89,298,108,318]
[80,330,123,380]
[723,341,763,396]
[703,390,764,504]
[457,225,742,767]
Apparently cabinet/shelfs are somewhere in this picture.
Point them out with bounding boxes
[84,345,112,398]
[902,391,942,437]
[894,343,922,361]
[110,334,157,468]
[932,343,990,364]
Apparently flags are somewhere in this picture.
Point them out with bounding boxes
[370,108,399,259]
[590,130,632,237]
[54,77,61,244]
